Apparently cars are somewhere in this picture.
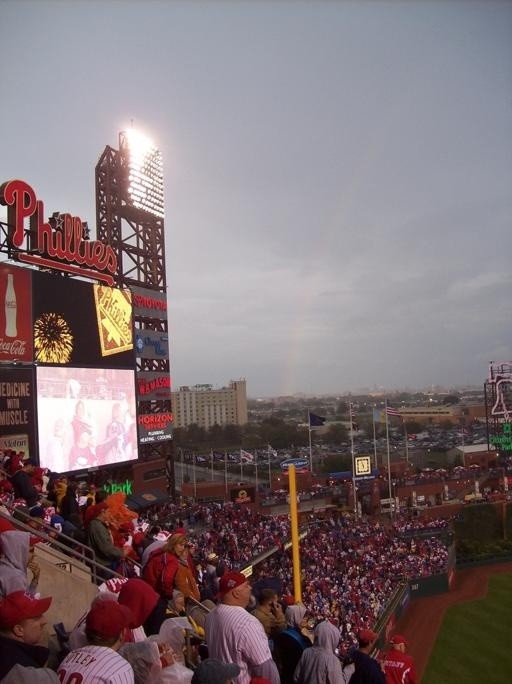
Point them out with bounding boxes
[482,488,505,503]
[262,484,337,508]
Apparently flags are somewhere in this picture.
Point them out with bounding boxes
[351,404,359,416]
[373,409,391,424]
[387,400,405,422]
[309,413,327,427]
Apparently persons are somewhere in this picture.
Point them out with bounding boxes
[417,534,449,577]
[0,447,419,683]
[418,470,449,533]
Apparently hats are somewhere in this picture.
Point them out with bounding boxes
[1,590,52,626]
[191,658,240,684]
[86,600,133,636]
[214,572,246,598]
[357,630,378,643]
[0,517,42,545]
[390,634,408,644]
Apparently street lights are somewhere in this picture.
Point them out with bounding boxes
[392,482,396,499]
